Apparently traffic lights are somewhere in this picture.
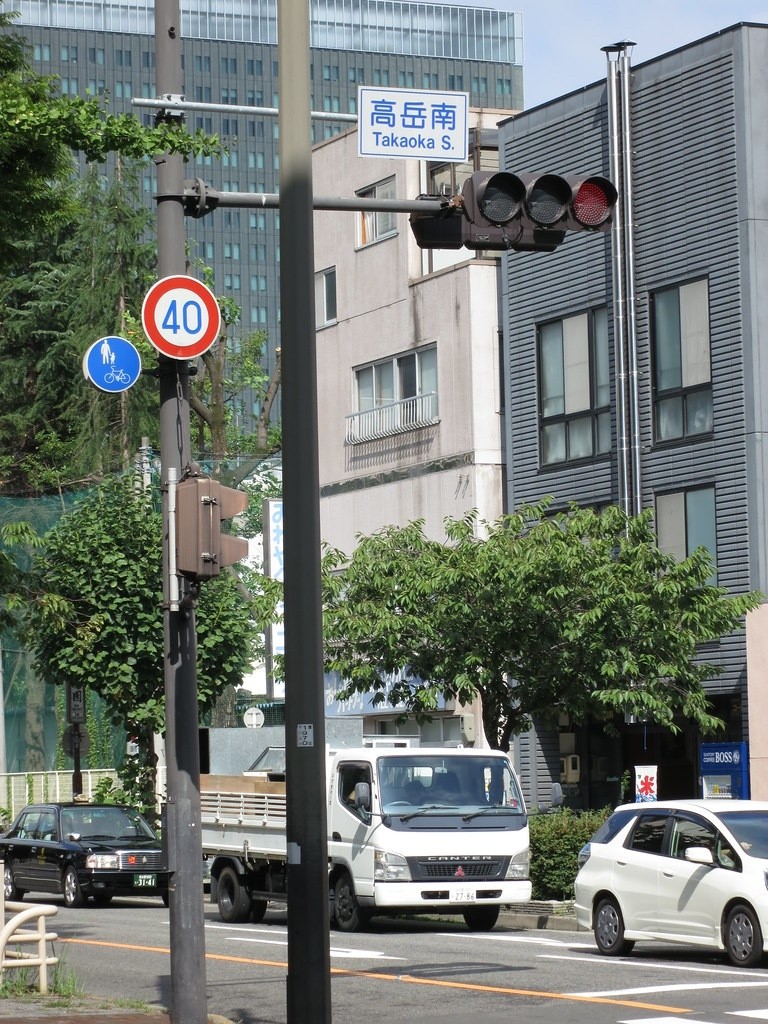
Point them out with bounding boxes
[123,719,145,756]
[467,169,619,233]
[218,482,248,569]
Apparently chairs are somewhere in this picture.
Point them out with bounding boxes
[432,771,468,805]
[52,815,72,833]
[341,769,370,805]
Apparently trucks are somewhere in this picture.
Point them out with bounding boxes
[200,744,532,932]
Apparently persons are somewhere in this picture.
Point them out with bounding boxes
[717,831,752,857]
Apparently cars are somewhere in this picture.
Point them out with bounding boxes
[0,802,170,909]
[573,799,768,967]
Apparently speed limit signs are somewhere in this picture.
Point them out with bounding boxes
[142,276,222,359]
